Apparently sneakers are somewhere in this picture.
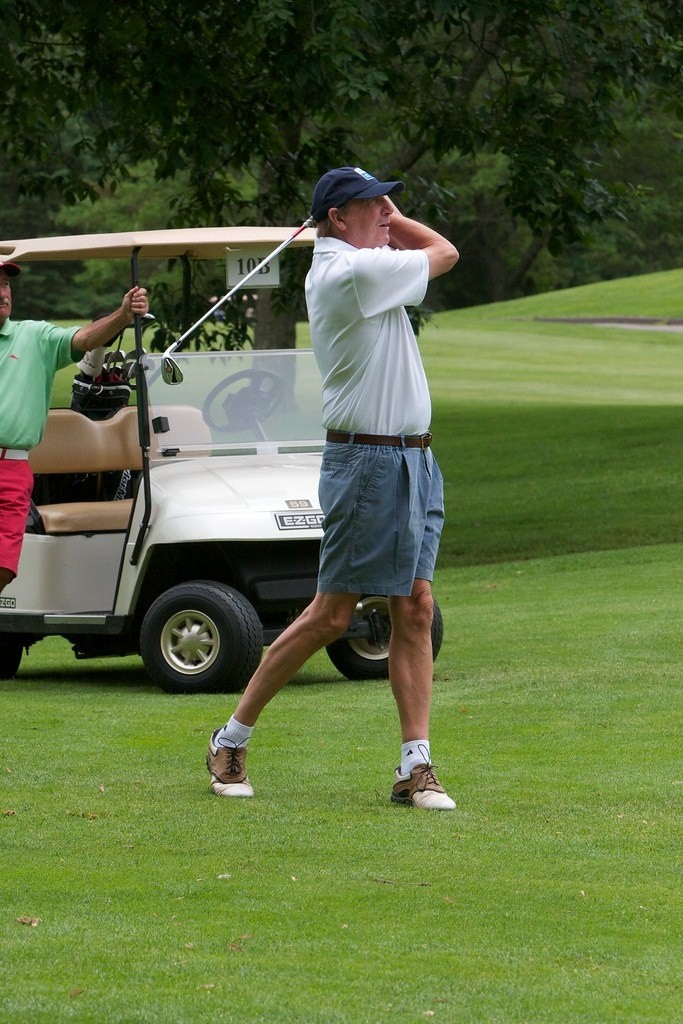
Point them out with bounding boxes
[204,727,255,798]
[390,744,456,810]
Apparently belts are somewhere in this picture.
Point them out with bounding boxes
[325,432,433,451]
[0,448,29,460]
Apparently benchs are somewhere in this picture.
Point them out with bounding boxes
[22,404,212,538]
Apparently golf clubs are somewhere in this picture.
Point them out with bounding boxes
[104,313,157,382]
[160,215,316,385]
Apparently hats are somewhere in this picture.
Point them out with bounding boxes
[0,262,22,277]
[309,166,405,220]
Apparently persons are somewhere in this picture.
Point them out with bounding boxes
[206,167,460,810]
[0,261,148,595]
